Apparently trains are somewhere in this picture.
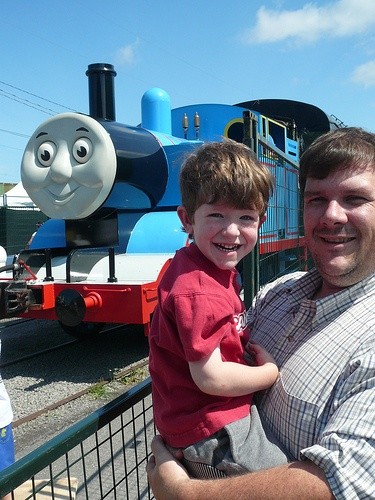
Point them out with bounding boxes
[5,63,349,343]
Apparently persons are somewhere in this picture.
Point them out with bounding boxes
[144,127,374,500]
[25,220,43,250]
[149,140,292,479]
[0,337,17,500]
